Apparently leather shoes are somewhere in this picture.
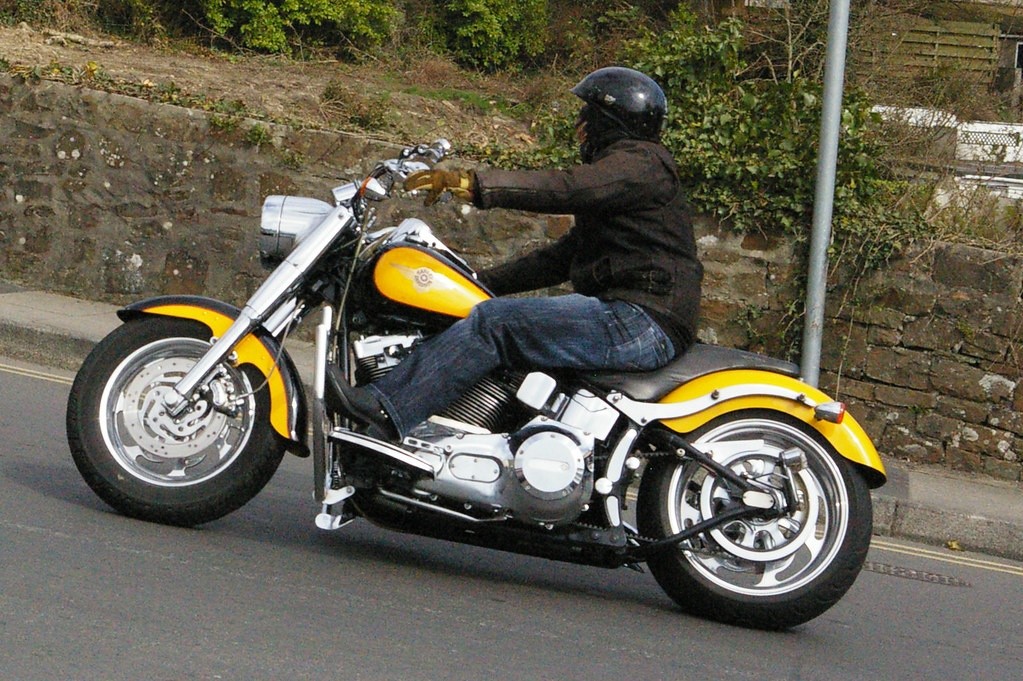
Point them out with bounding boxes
[325,363,399,445]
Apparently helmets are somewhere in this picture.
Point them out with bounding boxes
[568,67,668,140]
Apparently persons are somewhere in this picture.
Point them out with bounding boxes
[326,67,703,446]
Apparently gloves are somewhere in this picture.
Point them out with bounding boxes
[403,168,475,207]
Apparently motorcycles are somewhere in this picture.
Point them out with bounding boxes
[65,134,889,630]
